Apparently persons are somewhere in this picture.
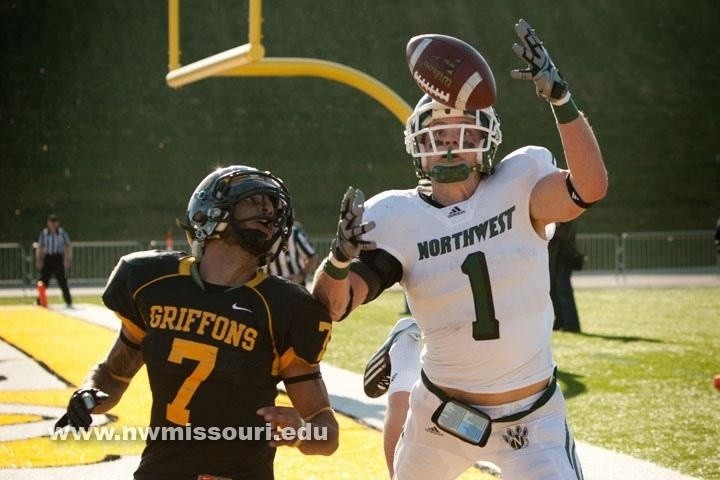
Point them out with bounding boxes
[310,18,609,479]
[257,221,319,288]
[52,165,340,479]
[547,216,583,333]
[35,214,76,309]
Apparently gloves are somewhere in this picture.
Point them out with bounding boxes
[330,186,377,262]
[54,387,110,431]
[510,19,569,101]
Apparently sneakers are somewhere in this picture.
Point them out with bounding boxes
[364,317,423,398]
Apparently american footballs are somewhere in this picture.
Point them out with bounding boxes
[406,34,496,110]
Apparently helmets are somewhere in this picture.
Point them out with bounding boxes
[404,91,502,182]
[186,165,293,264]
